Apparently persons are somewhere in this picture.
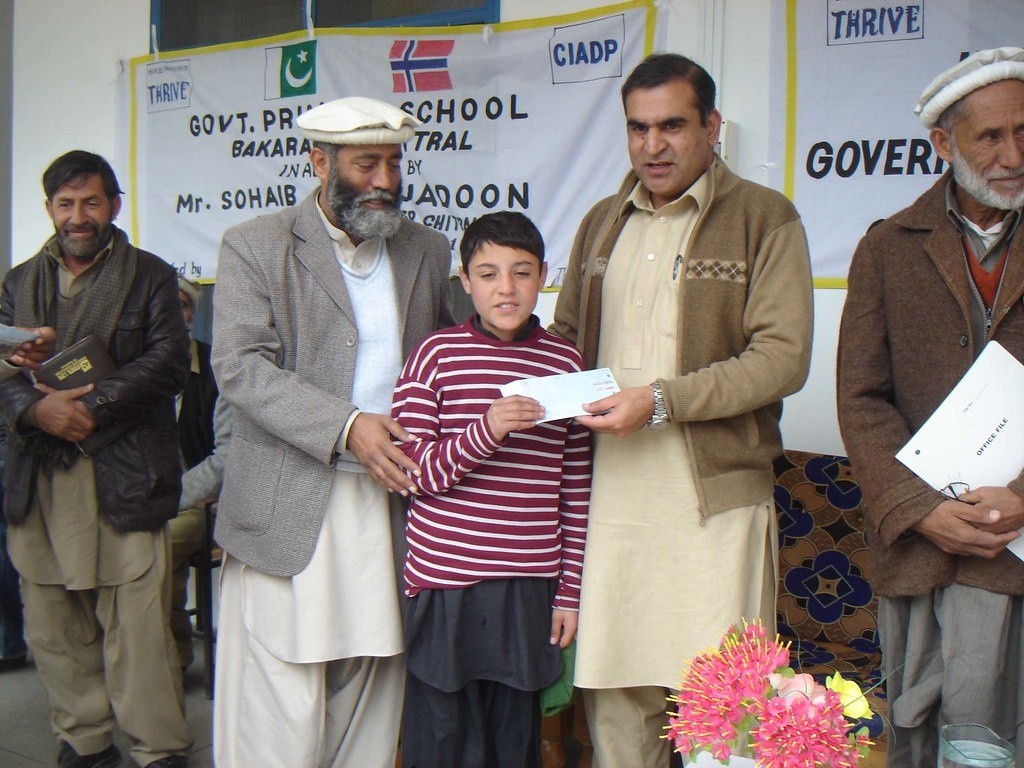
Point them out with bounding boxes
[169,276,236,679]
[1,321,57,373]
[546,52,815,768]
[206,96,457,768]
[385,209,594,768]
[0,149,196,768]
[834,46,1024,768]
[0,485,32,672]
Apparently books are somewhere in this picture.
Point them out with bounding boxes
[895,337,1024,563]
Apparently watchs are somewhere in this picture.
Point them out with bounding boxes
[646,381,671,433]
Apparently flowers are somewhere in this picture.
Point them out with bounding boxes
[659,615,909,768]
